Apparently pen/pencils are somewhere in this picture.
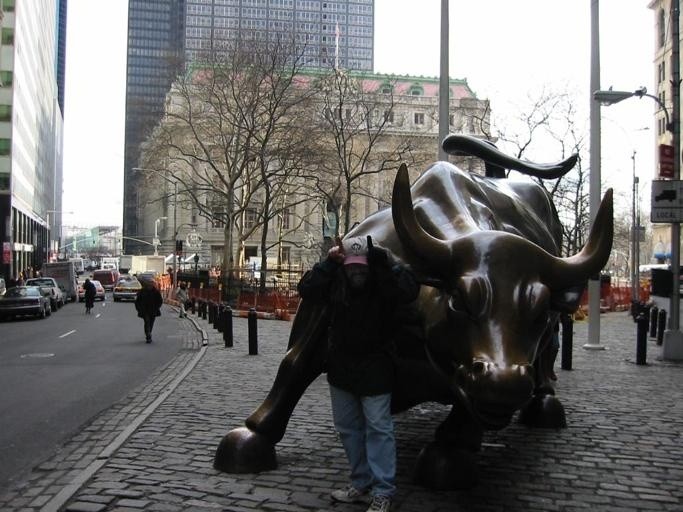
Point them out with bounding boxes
[89,270,119,291]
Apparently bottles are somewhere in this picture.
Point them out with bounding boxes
[341,236,369,267]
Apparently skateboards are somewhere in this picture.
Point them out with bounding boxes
[213,133,614,493]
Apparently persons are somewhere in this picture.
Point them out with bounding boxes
[82,277,96,315]
[175,280,188,320]
[134,277,162,344]
[295,233,423,512]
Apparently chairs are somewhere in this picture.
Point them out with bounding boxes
[366,495,392,512]
[329,483,373,504]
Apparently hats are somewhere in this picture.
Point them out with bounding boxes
[77,280,105,301]
[139,270,160,292]
[0,285,51,319]
[25,276,64,311]
[111,274,142,302]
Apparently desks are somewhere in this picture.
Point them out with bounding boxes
[592,86,683,362]
[132,167,179,300]
[44,211,74,264]
[151,216,167,256]
